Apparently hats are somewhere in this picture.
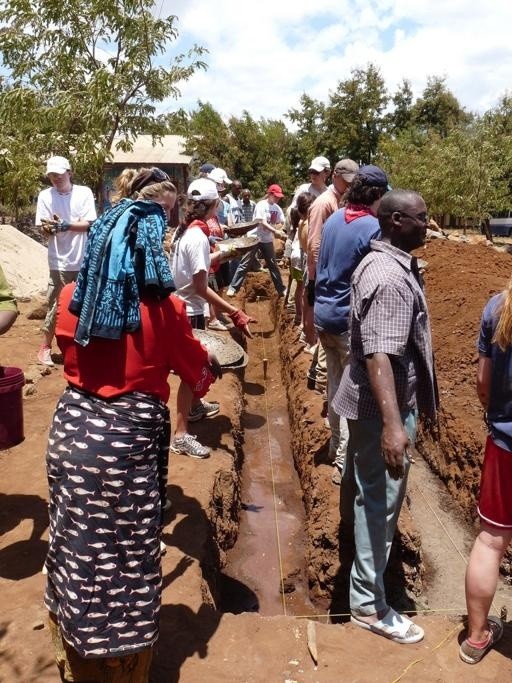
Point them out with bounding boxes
[200,163,233,192]
[266,184,284,198]
[308,156,330,173]
[334,158,360,183]
[46,155,71,178]
[356,164,392,191]
[187,178,220,203]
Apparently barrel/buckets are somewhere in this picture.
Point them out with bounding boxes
[0,367,25,450]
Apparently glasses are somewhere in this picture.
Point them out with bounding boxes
[151,167,171,181]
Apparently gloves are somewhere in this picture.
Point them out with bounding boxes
[213,243,241,265]
[227,308,259,340]
[274,229,288,242]
[40,213,70,239]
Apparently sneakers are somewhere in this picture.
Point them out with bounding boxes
[206,320,228,331]
[187,398,221,421]
[292,315,318,357]
[279,288,288,296]
[458,616,504,665]
[226,289,235,297]
[168,433,211,459]
[37,343,55,367]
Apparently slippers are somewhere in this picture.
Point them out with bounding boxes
[350,606,425,645]
